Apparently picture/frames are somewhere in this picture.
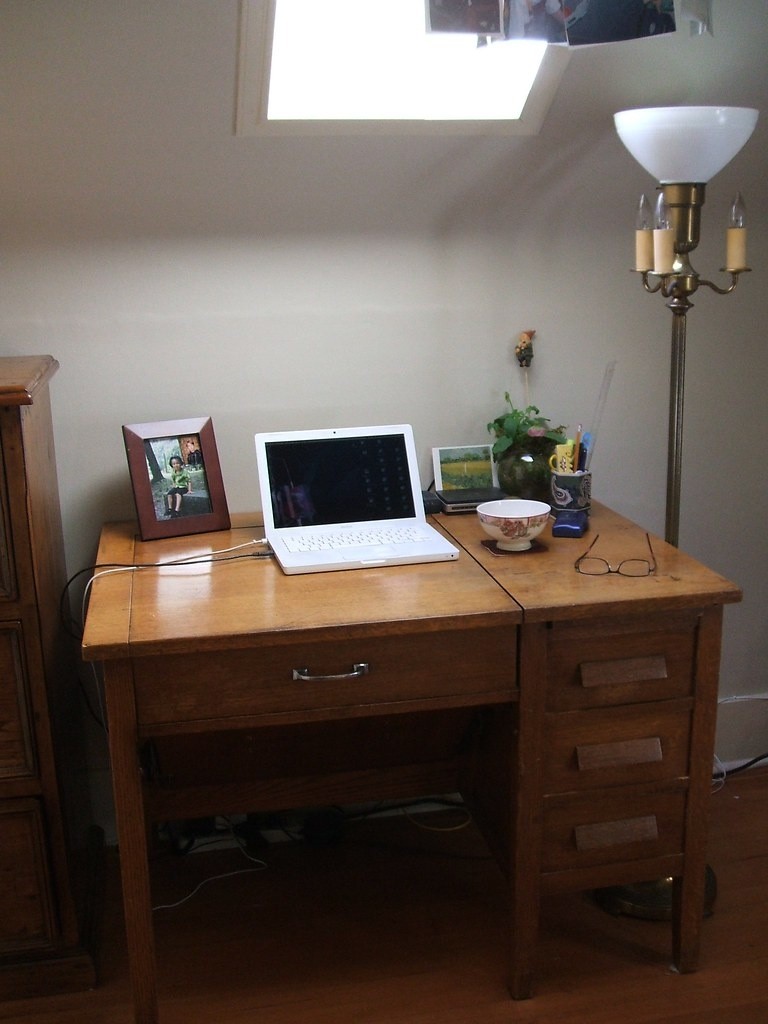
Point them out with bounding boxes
[122,417,231,540]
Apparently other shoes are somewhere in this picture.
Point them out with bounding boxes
[164,508,173,515]
[171,511,181,518]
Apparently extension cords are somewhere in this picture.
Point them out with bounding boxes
[187,817,307,855]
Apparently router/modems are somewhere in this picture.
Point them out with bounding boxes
[435,487,509,514]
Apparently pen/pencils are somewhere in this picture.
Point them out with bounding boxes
[574,424,582,473]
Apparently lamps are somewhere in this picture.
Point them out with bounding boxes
[589,105,760,923]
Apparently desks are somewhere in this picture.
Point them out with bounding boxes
[81,511,523,1024]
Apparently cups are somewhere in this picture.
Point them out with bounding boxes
[549,471,591,521]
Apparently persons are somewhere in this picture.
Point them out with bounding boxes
[164,456,195,518]
[186,440,203,465]
[515,330,536,368]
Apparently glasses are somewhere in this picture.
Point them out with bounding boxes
[574,532,657,578]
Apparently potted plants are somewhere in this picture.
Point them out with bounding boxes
[485,391,566,502]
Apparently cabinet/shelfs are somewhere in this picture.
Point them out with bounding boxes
[430,497,744,1000]
[0,355,107,999]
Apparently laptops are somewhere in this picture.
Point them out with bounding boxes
[253,423,459,575]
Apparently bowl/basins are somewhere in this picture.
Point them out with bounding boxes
[476,499,551,551]
